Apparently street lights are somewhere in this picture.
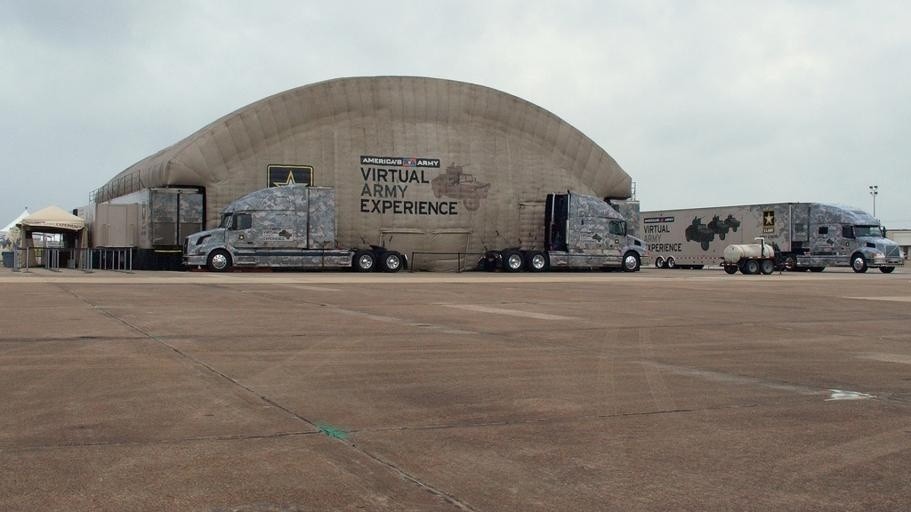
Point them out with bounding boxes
[868,185,879,218]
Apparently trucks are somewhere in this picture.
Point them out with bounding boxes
[181,182,409,273]
[640,201,906,273]
[486,188,650,272]
